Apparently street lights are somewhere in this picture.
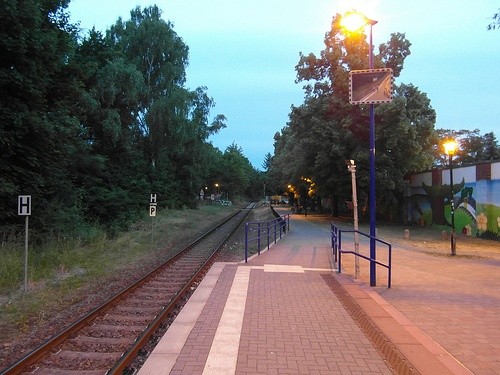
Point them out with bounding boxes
[440,135,460,256]
[338,10,395,289]
[213,184,220,195]
